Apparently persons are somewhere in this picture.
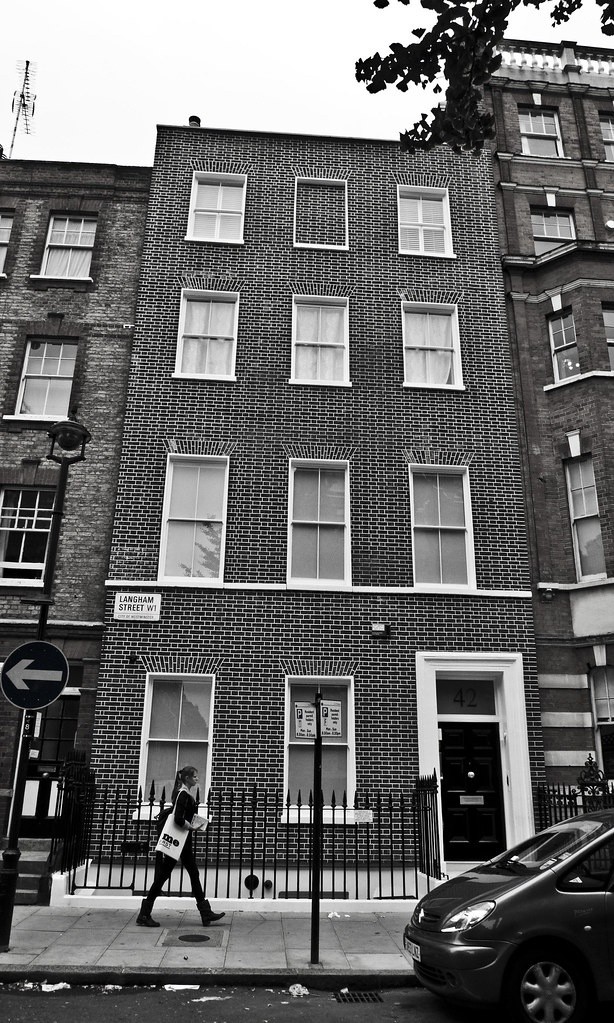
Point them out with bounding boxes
[137,766,225,927]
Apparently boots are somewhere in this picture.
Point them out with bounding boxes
[197,901,225,926]
[136,898,160,928]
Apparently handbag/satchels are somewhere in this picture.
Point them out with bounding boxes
[155,790,189,861]
[156,806,172,832]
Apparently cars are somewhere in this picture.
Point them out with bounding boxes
[404,809,614,1023]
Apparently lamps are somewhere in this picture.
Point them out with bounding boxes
[126,652,139,664]
[371,624,391,638]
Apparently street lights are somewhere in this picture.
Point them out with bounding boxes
[0,420,92,952]
